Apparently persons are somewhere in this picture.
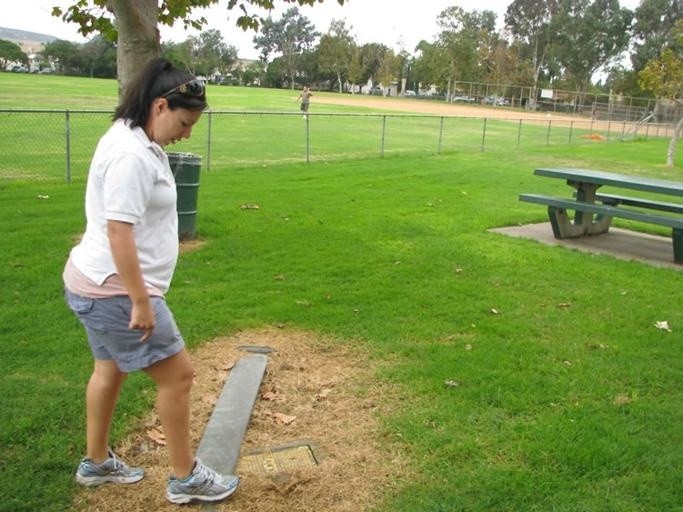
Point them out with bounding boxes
[294,86,313,120]
[57,53,241,506]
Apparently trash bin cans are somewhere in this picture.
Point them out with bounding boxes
[165,153,202,240]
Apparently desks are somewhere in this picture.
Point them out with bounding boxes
[530,167,682,265]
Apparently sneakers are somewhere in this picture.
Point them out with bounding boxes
[166,459,240,503]
[76,449,144,487]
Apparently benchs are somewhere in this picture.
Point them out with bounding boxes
[518,193,682,265]
[571,188,683,218]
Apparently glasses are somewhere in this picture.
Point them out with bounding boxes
[160,79,205,99]
